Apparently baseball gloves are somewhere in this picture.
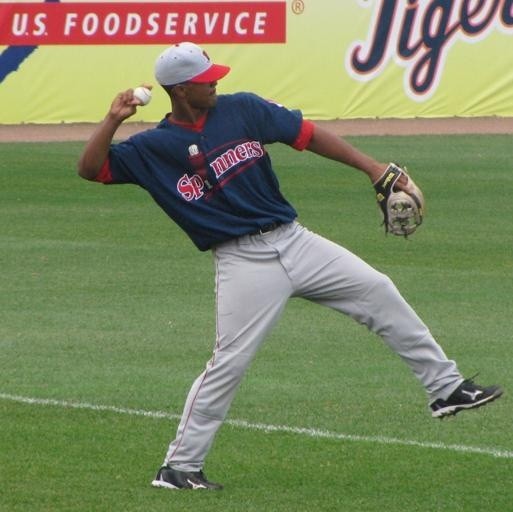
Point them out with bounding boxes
[372,162,424,242]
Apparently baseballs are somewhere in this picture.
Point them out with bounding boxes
[133,86,152,107]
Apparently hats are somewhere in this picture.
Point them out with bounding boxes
[155,42,230,85]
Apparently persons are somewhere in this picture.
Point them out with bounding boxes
[79,42,502,491]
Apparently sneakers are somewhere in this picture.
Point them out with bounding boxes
[430,373,503,418]
[152,467,223,489]
